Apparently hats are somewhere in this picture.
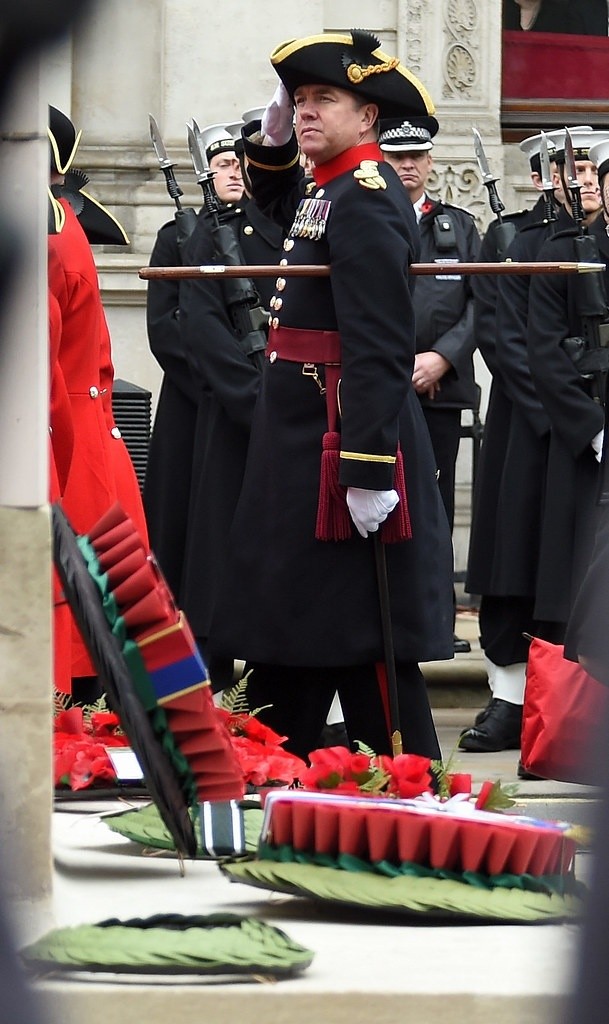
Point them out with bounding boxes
[49,106,82,174]
[225,122,248,157]
[242,108,266,124]
[519,126,592,170]
[589,139,609,182]
[59,169,131,246]
[378,116,439,151]
[548,131,609,164]
[270,28,435,115]
[201,123,235,159]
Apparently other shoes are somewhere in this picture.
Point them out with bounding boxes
[454,638,472,653]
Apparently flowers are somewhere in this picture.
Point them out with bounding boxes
[53,669,308,792]
[303,739,519,814]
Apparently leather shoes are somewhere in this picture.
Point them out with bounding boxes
[459,692,522,750]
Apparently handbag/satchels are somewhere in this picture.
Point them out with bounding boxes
[522,632,601,787]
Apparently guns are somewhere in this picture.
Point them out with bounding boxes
[184,122,271,371]
[471,126,518,262]
[538,129,561,223]
[190,117,222,207]
[147,111,198,260]
[562,126,609,506]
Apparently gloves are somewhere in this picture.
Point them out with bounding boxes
[262,80,293,146]
[346,487,399,538]
[591,428,604,462]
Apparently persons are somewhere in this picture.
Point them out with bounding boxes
[471,123,608,782]
[200,29,452,798]
[46,105,159,795]
[143,102,484,688]
